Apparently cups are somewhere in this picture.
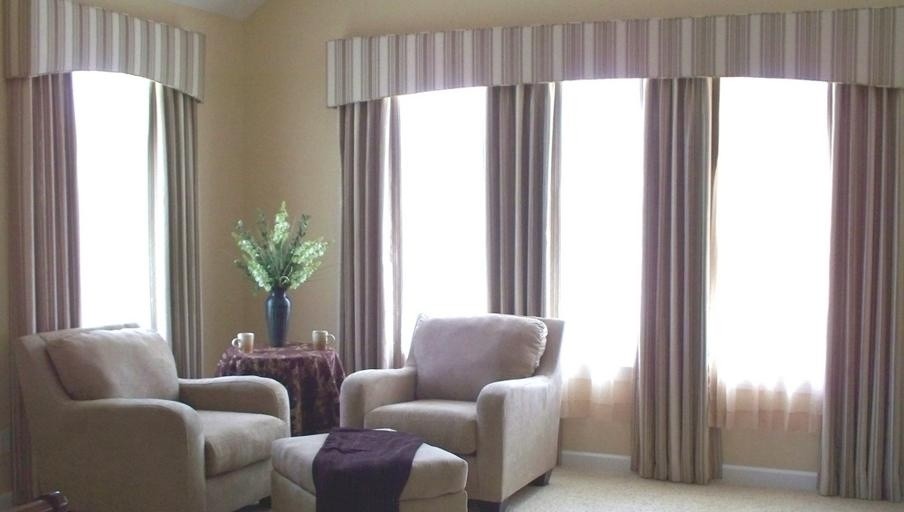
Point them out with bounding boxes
[311,330,335,351]
[231,332,255,355]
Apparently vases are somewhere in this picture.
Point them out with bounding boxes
[264,287,290,348]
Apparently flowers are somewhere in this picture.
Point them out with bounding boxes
[231,201,327,292]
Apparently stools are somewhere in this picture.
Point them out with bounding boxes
[270,428,469,512]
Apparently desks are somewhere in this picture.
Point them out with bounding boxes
[213,342,346,436]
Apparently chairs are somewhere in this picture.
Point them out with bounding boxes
[14,323,292,512]
[339,317,565,512]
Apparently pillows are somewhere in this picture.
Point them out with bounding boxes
[414,315,549,401]
[47,328,180,402]
